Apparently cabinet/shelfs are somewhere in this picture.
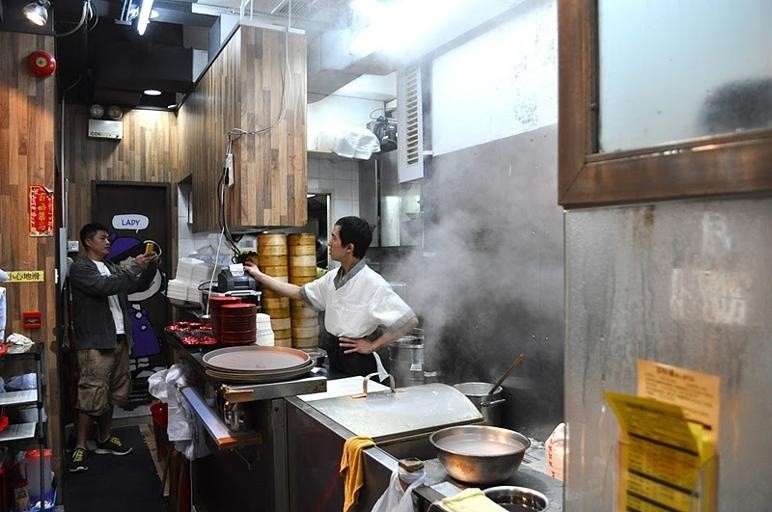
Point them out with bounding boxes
[0,341,46,511]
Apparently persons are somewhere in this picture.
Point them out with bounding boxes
[246,216,420,381]
[65,223,158,474]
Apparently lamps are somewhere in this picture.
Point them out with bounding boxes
[22,1,52,27]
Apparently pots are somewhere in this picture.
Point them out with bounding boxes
[388,336,423,388]
[453,382,507,427]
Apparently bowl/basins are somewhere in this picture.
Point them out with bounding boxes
[428,425,550,512]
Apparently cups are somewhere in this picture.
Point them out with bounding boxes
[27,450,53,498]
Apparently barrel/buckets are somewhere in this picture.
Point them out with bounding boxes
[209,293,242,339]
[222,303,256,343]
[21,447,52,499]
[150,402,168,461]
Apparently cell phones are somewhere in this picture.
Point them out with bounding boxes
[145,243,155,254]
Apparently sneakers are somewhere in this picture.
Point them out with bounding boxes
[69,447,88,472]
[94,435,133,455]
